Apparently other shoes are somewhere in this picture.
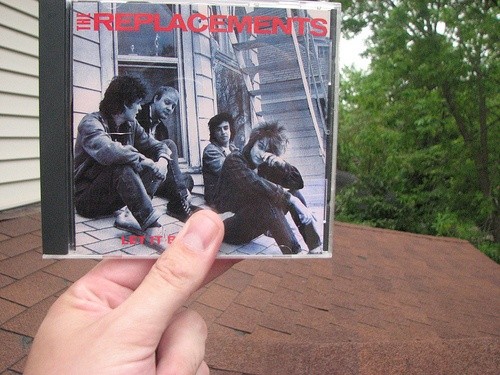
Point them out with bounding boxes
[143,223,173,253]
[188,203,202,211]
[166,197,193,223]
[113,210,146,236]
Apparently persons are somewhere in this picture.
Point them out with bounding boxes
[75,75,321,255]
[20,209,246,375]
